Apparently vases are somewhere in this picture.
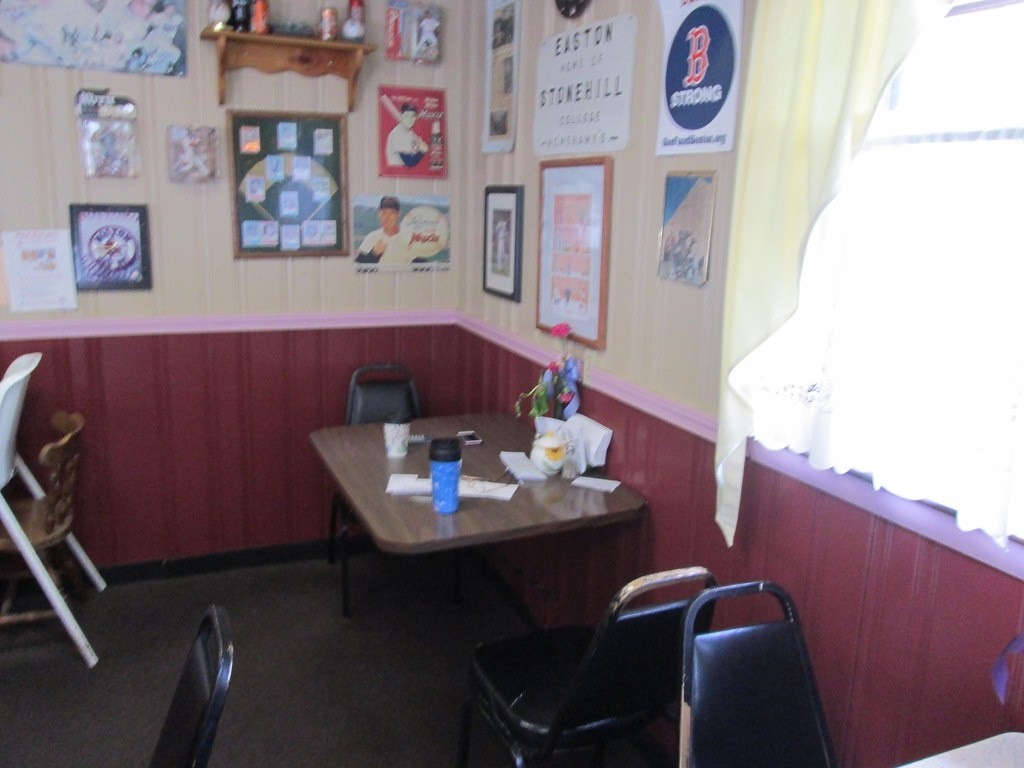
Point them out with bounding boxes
[554,401,568,421]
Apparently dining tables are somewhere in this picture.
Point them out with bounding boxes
[309,413,646,634]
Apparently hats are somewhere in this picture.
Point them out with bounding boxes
[380,196,399,208]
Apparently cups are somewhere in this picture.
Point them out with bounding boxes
[382,410,412,460]
[429,437,463,514]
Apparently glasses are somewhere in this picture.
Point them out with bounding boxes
[462,466,514,493]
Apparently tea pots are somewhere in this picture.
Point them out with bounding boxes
[530,431,576,477]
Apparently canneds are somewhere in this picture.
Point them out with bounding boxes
[319,7,337,41]
[250,0,270,34]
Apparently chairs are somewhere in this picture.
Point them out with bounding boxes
[329,364,426,616]
[456,566,719,768]
[679,580,838,768]
[0,413,87,629]
[149,603,234,768]
[0,351,107,669]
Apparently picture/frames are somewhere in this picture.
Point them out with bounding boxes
[226,108,352,259]
[70,204,152,290]
[536,156,613,349]
[482,185,524,301]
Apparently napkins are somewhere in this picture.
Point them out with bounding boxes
[565,413,613,468]
[384,472,431,496]
[570,477,621,493]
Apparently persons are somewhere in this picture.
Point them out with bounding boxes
[419,9,439,50]
[385,103,428,168]
[355,195,426,263]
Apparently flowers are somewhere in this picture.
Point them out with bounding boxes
[514,321,581,420]
